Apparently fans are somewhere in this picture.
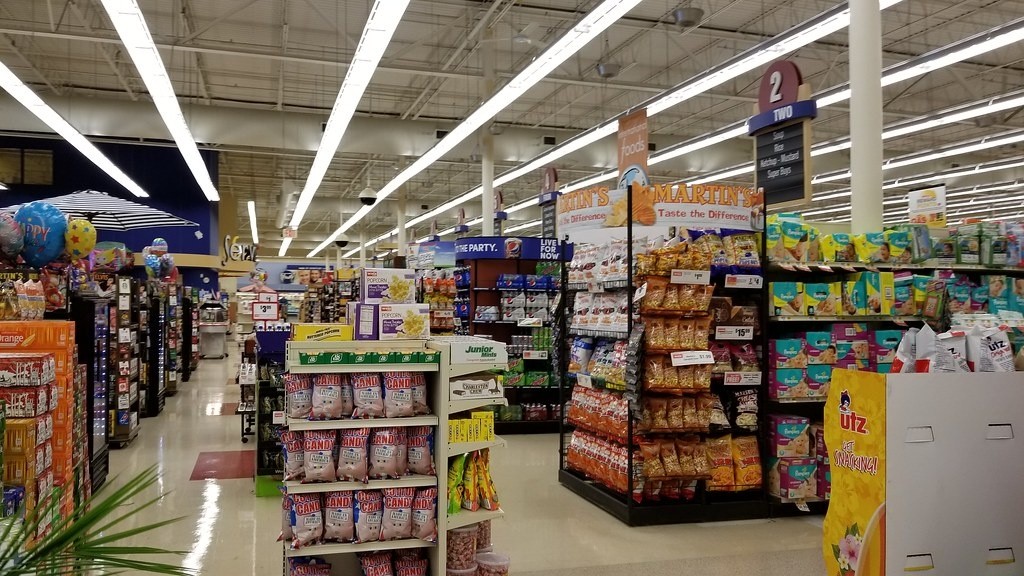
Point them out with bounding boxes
[479,3,548,50]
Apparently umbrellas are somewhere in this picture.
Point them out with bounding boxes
[0,189,201,232]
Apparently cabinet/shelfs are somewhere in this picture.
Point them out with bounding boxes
[551,183,1024,526]
[234,239,360,498]
[454,238,575,435]
[0,264,201,576]
[282,336,509,576]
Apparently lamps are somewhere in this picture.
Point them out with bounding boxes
[307,1,1024,257]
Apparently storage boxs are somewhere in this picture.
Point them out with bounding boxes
[289,322,354,341]
[346,301,360,325]
[431,336,508,365]
[474,273,560,322]
[379,303,430,340]
[769,271,907,500]
[356,302,380,340]
[449,372,503,401]
[361,268,417,305]
[449,410,495,442]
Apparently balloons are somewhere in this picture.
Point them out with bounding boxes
[142,238,178,282]
[13,202,67,272]
[63,219,98,274]
[0,212,24,263]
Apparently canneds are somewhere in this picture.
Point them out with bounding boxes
[475,274,561,354]
